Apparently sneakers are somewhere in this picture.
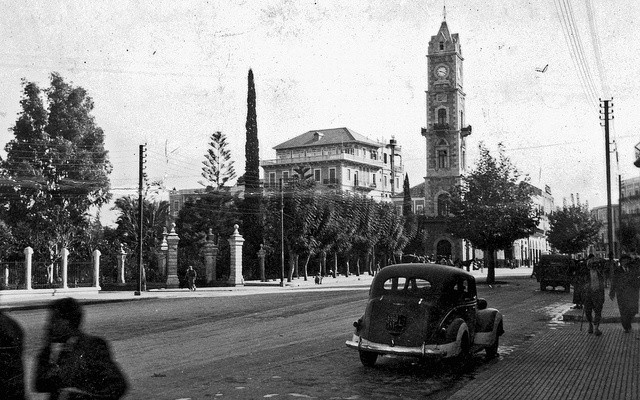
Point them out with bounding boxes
[588,324,594,332]
[594,328,601,335]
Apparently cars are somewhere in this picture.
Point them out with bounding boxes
[345,262,504,378]
[535,253,572,291]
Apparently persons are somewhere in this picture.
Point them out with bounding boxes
[609,253,639,330]
[583,258,605,335]
[30,298,128,399]
[0,308,27,398]
[185,265,197,291]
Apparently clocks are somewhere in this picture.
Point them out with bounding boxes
[434,63,450,80]
